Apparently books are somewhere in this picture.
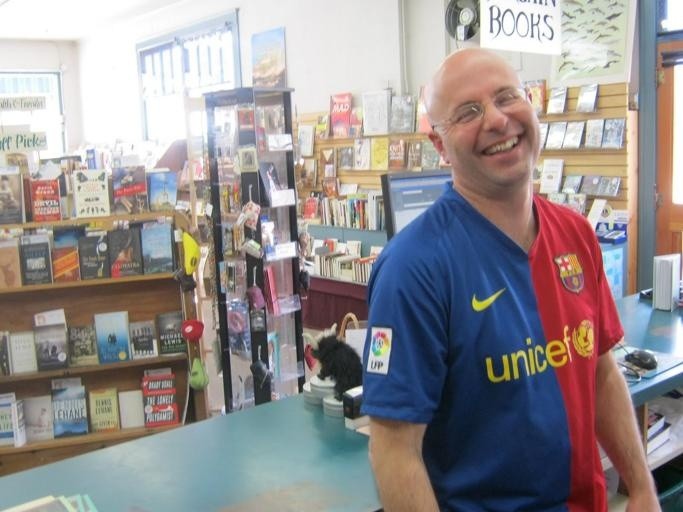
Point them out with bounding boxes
[296,178,387,230]
[1,167,178,225]
[300,140,444,188]
[1,307,189,375]
[4,491,99,512]
[538,159,629,249]
[0,367,181,448]
[1,216,177,289]
[537,119,625,150]
[647,409,671,457]
[297,85,433,156]
[522,80,599,114]
[299,232,387,286]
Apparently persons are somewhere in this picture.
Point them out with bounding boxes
[361,48,661,512]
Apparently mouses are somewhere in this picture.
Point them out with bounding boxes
[625,350,657,369]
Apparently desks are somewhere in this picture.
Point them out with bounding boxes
[1,290,683,512]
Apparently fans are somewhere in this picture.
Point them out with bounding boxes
[444,0,480,42]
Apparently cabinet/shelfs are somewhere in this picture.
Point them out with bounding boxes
[0,210,211,479]
[202,88,307,413]
[294,83,630,232]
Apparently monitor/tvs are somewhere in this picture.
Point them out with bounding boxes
[381,169,455,242]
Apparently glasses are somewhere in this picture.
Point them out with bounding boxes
[430,86,525,134]
